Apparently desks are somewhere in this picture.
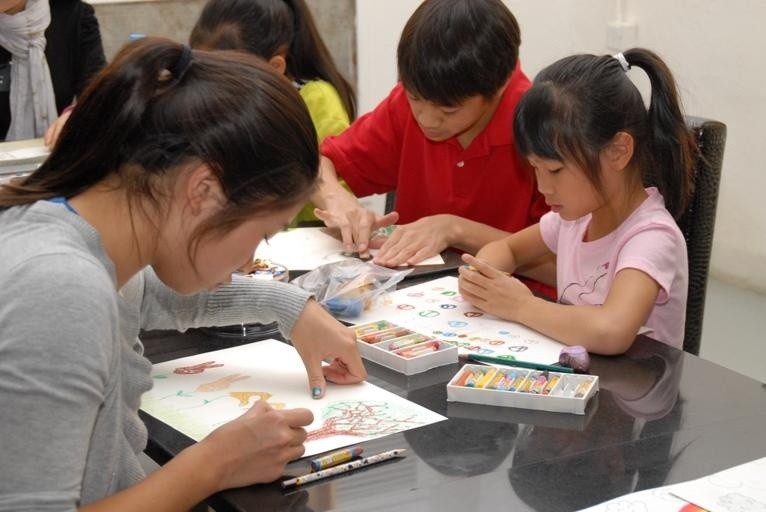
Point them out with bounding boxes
[144,219,761,511]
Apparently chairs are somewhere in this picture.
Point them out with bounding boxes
[644,111,725,355]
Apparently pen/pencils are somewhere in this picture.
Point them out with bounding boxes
[282,447,407,489]
[455,354,592,398]
[355,320,441,357]
[464,265,510,277]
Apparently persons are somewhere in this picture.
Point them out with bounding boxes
[0,0,108,152]
[189,0,357,228]
[507,335,697,512]
[309,0,558,303]
[0,36,366,512]
[459,48,713,356]
